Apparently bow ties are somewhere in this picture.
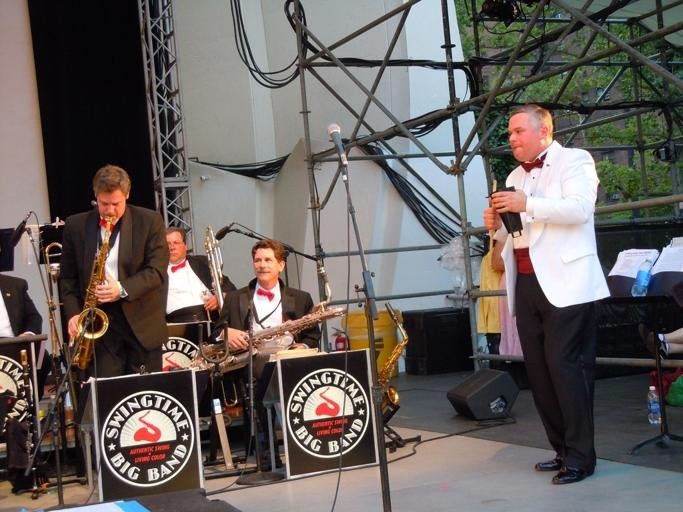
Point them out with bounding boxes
[521,158,544,172]
[171,262,185,273]
[257,288,275,302]
[98,219,115,232]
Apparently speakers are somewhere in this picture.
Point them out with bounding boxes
[446,366,520,421]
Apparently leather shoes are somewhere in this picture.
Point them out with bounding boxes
[535,455,596,484]
[255,432,282,471]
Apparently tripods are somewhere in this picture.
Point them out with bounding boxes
[16,258,87,498]
[629,315,683,456]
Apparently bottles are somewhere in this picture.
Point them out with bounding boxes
[646,386,662,424]
[632,260,652,296]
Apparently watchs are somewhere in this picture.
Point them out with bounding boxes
[119,283,128,299]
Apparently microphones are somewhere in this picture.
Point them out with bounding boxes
[8,212,31,248]
[215,223,233,240]
[326,123,348,166]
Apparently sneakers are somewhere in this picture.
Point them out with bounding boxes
[639,323,669,360]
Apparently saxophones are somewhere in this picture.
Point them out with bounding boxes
[191,307,348,377]
[68,215,112,371]
[377,302,409,425]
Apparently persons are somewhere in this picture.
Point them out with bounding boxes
[484,104,611,485]
[60,164,169,478]
[477,238,504,370]
[640,324,683,359]
[206,240,322,471]
[0,274,51,494]
[491,221,523,363]
[164,227,236,344]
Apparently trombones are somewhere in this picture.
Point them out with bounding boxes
[204,226,225,316]
[45,243,63,359]
